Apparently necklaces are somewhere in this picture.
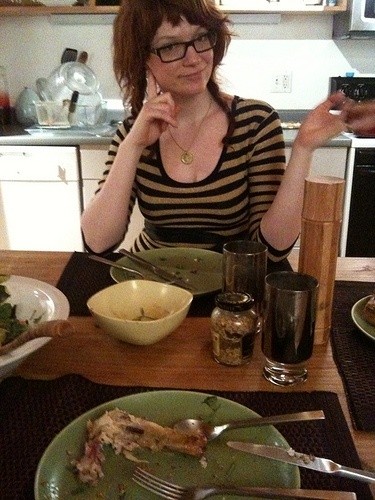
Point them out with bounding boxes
[168,96,214,164]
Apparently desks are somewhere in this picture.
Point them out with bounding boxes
[0,250,375,500]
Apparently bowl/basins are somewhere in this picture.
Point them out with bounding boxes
[47,62,104,120]
[86,278,194,347]
[0,273,71,378]
[16,87,44,126]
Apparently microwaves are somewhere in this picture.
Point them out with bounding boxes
[332,0,375,38]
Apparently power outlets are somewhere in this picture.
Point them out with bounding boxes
[269,71,292,93]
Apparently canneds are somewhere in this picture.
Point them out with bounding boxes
[211,291,259,367]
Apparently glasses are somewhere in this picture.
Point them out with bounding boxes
[149,30,217,64]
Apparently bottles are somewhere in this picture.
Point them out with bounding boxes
[0,65,13,128]
[211,292,260,366]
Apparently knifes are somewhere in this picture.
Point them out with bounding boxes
[116,247,198,292]
[224,440,375,482]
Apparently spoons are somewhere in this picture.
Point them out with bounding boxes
[174,409,326,443]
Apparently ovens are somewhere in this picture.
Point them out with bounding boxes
[340,135,375,259]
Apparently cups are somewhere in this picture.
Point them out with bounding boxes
[222,239,268,333]
[262,270,320,388]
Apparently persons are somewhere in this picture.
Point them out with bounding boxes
[80,1,375,264]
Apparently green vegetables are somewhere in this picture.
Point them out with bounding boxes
[0,285,45,339]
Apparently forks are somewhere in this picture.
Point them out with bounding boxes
[88,254,175,285]
[130,466,356,500]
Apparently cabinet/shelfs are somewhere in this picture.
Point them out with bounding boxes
[0,146,352,257]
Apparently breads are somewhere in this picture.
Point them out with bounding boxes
[364,296,375,326]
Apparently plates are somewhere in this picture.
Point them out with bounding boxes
[349,295,375,339]
[109,247,225,297]
[35,390,302,499]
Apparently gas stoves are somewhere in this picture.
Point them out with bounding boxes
[328,77,375,138]
